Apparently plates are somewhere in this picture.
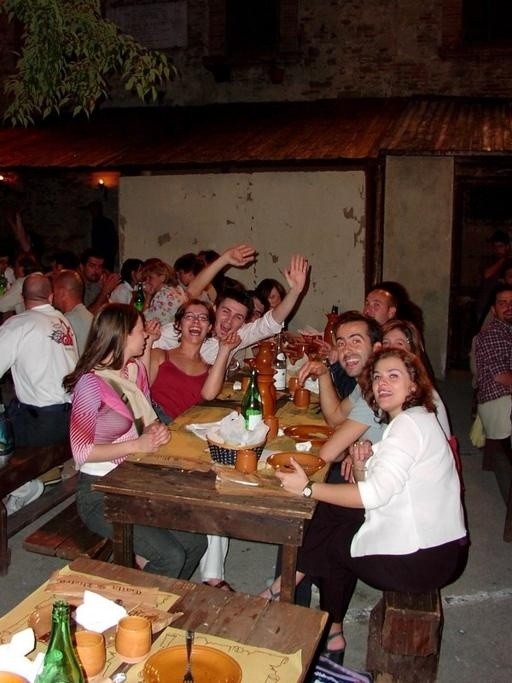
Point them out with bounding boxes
[284,424,339,446]
[267,452,326,476]
[143,644,242,682]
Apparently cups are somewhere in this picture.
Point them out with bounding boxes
[66,631,109,679]
[115,613,153,659]
[236,449,259,475]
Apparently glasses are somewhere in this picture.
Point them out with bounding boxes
[182,310,213,323]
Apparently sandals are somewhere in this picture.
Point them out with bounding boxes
[202,576,239,595]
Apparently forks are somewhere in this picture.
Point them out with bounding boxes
[183,629,194,682]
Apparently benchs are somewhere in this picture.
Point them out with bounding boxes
[364,587,447,682]
[1,438,83,572]
[478,436,510,547]
[20,495,117,562]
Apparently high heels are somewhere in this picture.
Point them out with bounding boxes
[327,621,347,667]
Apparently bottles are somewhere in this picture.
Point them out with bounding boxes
[32,599,88,682]
[133,281,145,312]
[241,304,340,441]
